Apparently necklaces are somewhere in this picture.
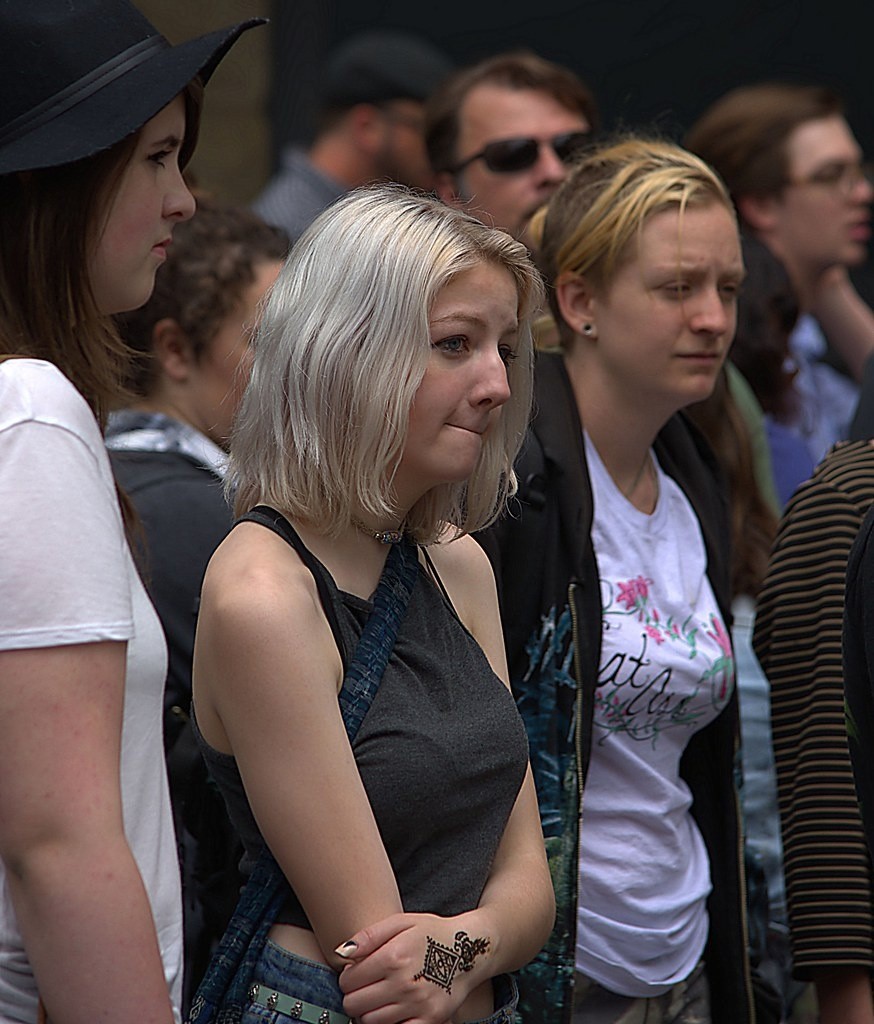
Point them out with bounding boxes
[345,510,406,544]
[626,452,649,498]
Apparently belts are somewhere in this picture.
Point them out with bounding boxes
[244,979,355,1024]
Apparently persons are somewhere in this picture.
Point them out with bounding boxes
[98,206,293,1024]
[473,140,782,1024]
[190,186,557,1024]
[684,85,874,1024]
[0,0,270,1024]
[423,55,609,243]
[250,35,452,248]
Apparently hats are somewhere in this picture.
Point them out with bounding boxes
[1,0,266,173]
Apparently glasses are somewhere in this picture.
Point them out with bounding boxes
[457,132,592,177]
[786,162,874,197]
